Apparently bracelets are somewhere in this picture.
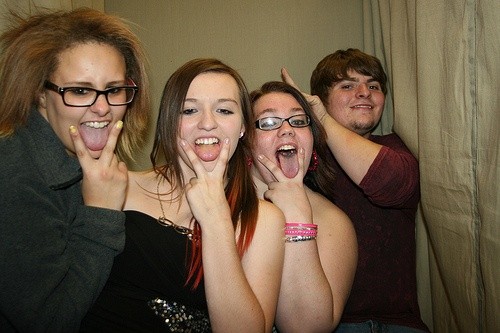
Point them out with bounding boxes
[285,223,318,242]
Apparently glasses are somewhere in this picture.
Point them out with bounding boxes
[254,114,312,131]
[43,77,139,107]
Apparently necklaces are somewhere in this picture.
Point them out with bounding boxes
[157,174,191,236]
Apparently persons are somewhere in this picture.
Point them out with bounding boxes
[78,58,287,333]
[281,48,428,333]
[244,81,359,333]
[0,1,151,333]
